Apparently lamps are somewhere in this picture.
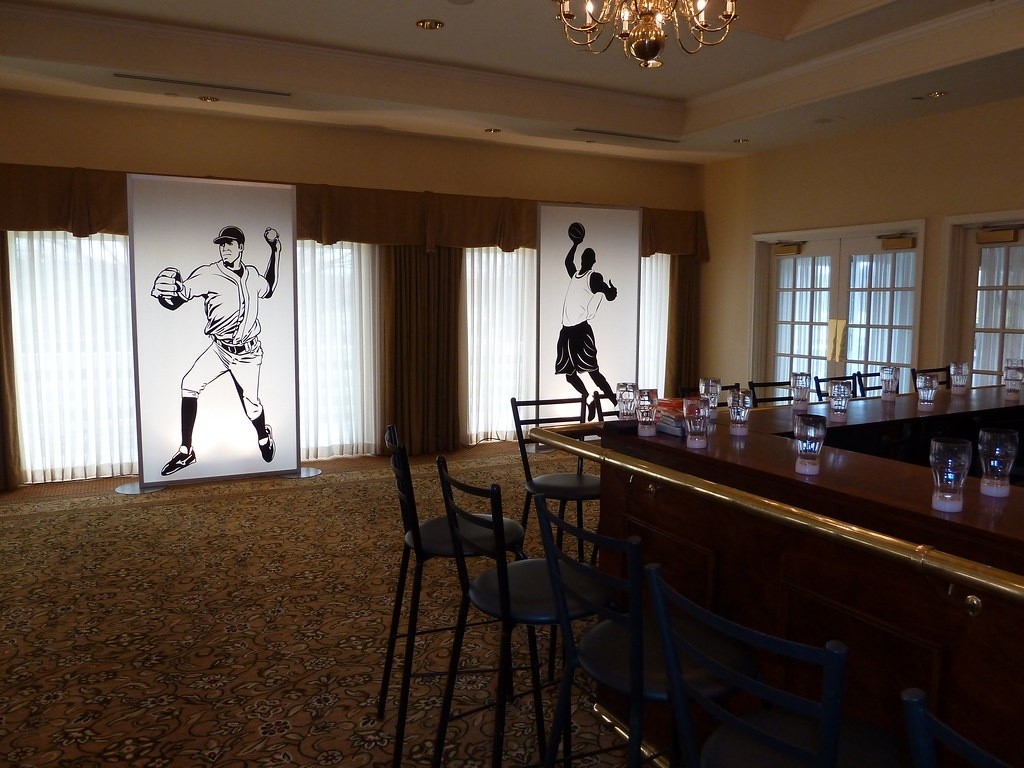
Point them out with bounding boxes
[552,0,739,67]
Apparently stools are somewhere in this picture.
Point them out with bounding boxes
[432,453,620,767]
[534,493,756,767]
[645,560,914,768]
[511,393,601,564]
[681,365,953,411]
[901,686,1013,768]
[594,392,619,422]
[377,424,546,768]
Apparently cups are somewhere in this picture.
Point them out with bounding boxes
[633,389,658,436]
[793,414,827,475]
[880,367,899,402]
[930,438,972,512]
[726,388,751,435]
[829,381,852,423]
[699,377,721,419]
[616,382,638,420]
[917,374,938,412]
[977,427,1019,496]
[683,398,710,449]
[1003,366,1024,399]
[1006,358,1024,366]
[791,372,811,414]
[950,362,968,394]
